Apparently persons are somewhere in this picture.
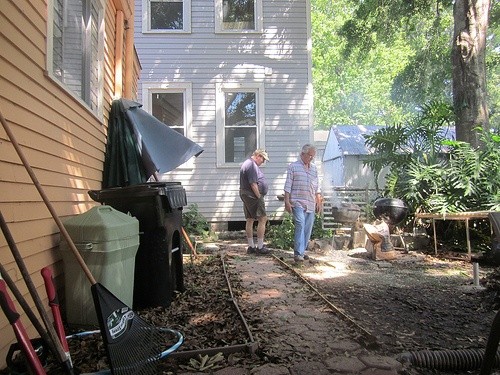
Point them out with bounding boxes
[239,148,270,255]
[284,144,321,261]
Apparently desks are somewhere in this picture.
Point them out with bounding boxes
[416,211,489,263]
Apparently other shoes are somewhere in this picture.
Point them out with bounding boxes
[294,254,309,266]
[247,245,256,254]
[255,247,273,255]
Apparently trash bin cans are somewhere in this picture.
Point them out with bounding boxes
[58,204,140,327]
[87,181,187,312]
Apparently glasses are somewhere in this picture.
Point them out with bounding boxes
[309,155,316,158]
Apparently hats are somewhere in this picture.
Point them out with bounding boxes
[253,148,270,162]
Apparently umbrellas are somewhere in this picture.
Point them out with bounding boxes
[102,99,204,258]
